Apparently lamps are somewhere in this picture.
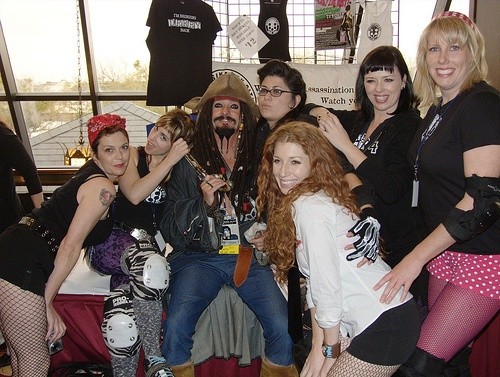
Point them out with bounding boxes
[56,0,96,167]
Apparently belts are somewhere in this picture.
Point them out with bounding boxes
[17,216,60,255]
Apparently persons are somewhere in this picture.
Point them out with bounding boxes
[259,121,420,377]
[0,113,129,377]
[155,73,299,377]
[85,109,196,377]
[256,60,380,267]
[0,121,45,234]
[306,45,423,304]
[373,10,500,377]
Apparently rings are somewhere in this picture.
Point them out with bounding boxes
[255,230,262,236]
[210,184,213,188]
[207,182,210,185]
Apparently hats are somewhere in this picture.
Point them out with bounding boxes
[86,114,126,147]
[193,71,258,118]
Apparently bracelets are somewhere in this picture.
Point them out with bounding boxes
[322,339,341,358]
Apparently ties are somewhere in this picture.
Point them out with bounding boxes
[288,261,303,343]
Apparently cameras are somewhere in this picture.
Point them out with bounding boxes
[48,331,63,355]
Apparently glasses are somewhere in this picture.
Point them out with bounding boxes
[258,88,294,97]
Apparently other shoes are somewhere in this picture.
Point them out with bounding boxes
[143,361,174,377]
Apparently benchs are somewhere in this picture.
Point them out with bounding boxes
[44,251,303,376]
